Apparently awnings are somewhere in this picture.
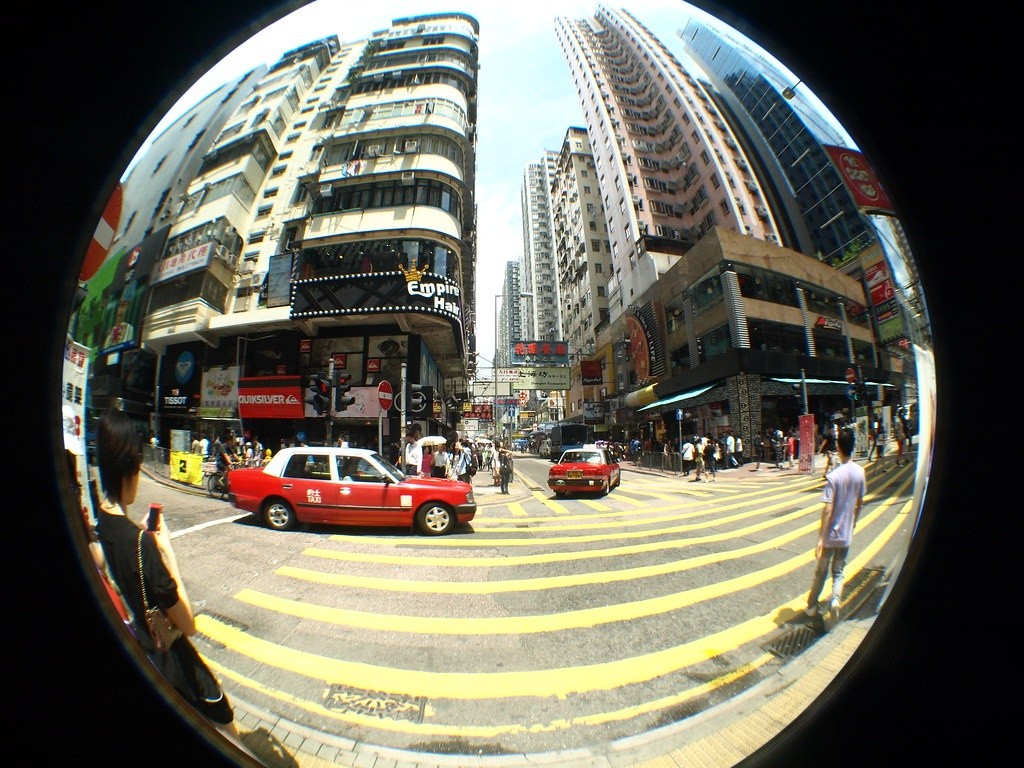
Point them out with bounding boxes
[637,384,719,412]
[771,377,895,387]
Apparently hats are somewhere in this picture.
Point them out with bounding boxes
[246,442,251,446]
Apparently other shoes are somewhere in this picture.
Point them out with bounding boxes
[755,466,762,471]
[696,474,701,481]
[832,600,839,622]
[219,478,225,486]
[806,606,818,616]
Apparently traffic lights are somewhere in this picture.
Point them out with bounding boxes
[335,370,355,412]
[406,382,422,415]
[858,381,867,399]
[852,386,861,408]
[313,379,332,412]
[304,371,325,410]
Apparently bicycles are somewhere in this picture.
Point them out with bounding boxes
[208,462,244,499]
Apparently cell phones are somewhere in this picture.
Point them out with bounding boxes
[147,503,163,532]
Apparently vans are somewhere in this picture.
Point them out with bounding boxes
[896,403,919,435]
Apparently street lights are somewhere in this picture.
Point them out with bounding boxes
[495,292,533,443]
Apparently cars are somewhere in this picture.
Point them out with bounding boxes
[547,444,620,496]
[227,442,476,537]
[546,442,551,458]
[512,440,529,451]
[539,440,548,458]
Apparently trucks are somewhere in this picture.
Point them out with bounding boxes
[550,424,588,461]
[86,405,107,453]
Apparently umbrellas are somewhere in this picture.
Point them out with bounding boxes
[417,436,447,450]
[478,439,493,443]
[463,438,473,443]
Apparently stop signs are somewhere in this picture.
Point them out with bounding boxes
[78,182,125,285]
[376,380,393,411]
[846,368,855,383]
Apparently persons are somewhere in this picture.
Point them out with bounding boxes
[301,434,357,481]
[754,410,912,474]
[97,407,236,737]
[104,300,133,348]
[807,428,867,628]
[191,429,272,486]
[600,433,743,483]
[151,434,160,449]
[397,433,512,494]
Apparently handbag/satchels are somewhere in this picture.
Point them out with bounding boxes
[468,466,476,475]
[139,530,183,653]
[682,453,684,458]
[695,454,703,462]
